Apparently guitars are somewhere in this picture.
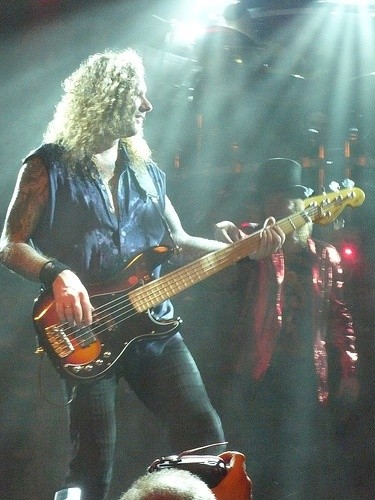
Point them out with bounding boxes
[31,178,366,388]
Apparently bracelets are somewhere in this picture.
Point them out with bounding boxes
[39,259,71,287]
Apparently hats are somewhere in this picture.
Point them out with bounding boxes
[249,158,314,200]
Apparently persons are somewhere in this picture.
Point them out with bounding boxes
[0,47,286,500]
[214,158,360,500]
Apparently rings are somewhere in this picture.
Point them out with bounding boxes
[64,304,72,309]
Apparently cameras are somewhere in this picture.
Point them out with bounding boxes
[148,441,228,490]
